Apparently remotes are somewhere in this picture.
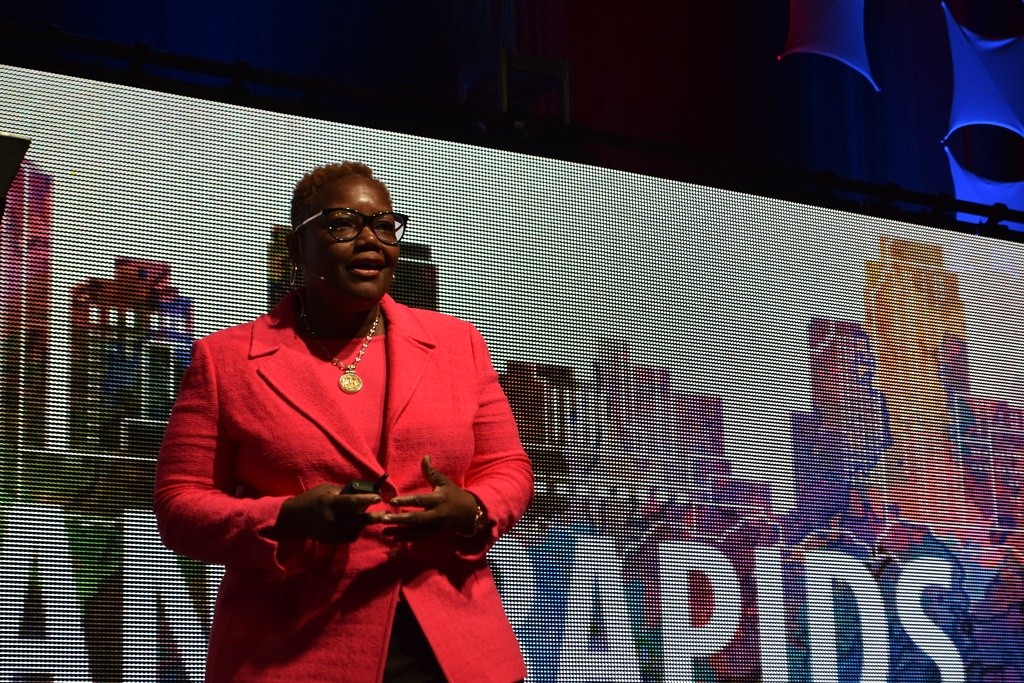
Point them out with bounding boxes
[339,474,389,496]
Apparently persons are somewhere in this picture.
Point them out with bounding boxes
[152,162,534,683]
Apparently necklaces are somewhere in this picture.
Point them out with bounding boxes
[300,294,381,393]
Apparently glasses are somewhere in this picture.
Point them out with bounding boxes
[293,207,409,246]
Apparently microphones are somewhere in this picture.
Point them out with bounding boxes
[301,264,324,280]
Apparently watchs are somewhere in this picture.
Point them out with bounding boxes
[473,495,486,527]
